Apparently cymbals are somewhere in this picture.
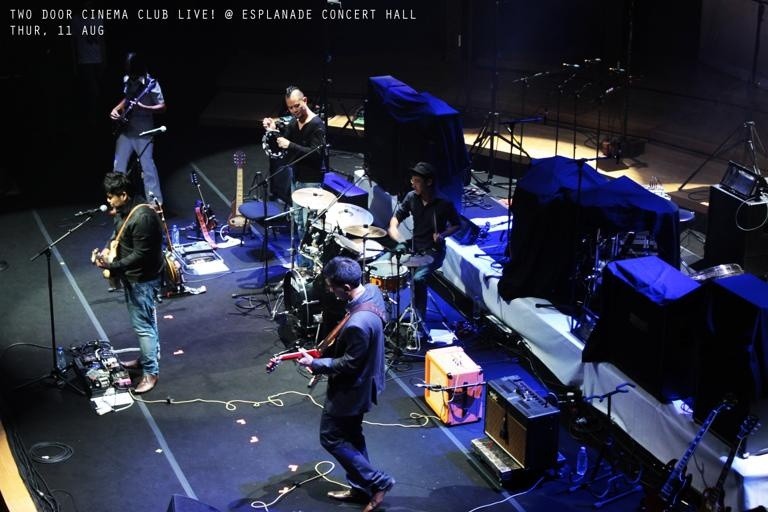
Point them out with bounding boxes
[342,223,388,238]
[391,251,436,268]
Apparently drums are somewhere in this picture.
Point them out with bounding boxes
[321,202,374,230]
[289,185,338,228]
[295,219,338,265]
[366,257,411,293]
[342,237,384,261]
[318,231,364,271]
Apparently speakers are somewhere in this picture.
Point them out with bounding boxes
[424,345,484,426]
[167,495,222,511]
[704,186,768,273]
[484,375,561,469]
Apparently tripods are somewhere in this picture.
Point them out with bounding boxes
[232,185,284,319]
[469,78,533,180]
[311,74,365,154]
[382,256,427,380]
[678,1,768,191]
[11,217,87,394]
[585,84,629,168]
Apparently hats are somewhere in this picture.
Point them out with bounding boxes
[408,162,436,179]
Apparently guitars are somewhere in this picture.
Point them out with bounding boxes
[641,391,738,509]
[90,245,124,293]
[695,412,761,510]
[109,75,158,138]
[227,149,252,237]
[266,334,337,375]
[189,168,221,251]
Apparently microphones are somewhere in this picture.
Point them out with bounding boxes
[518,115,547,124]
[74,204,108,217]
[563,63,580,68]
[321,137,328,160]
[511,71,550,84]
[139,125,167,137]
[584,58,601,63]
[598,88,616,98]
[609,68,625,73]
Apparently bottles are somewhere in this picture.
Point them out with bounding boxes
[56,346,67,374]
[577,446,587,475]
[172,225,179,246]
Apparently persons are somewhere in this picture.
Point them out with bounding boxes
[389,163,461,347]
[296,255,397,512]
[96,171,166,393]
[262,87,325,239]
[111,50,165,210]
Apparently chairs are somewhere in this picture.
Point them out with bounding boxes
[239,166,295,261]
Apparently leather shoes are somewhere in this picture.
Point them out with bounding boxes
[135,372,159,393]
[364,479,394,512]
[328,489,370,502]
[121,359,139,368]
